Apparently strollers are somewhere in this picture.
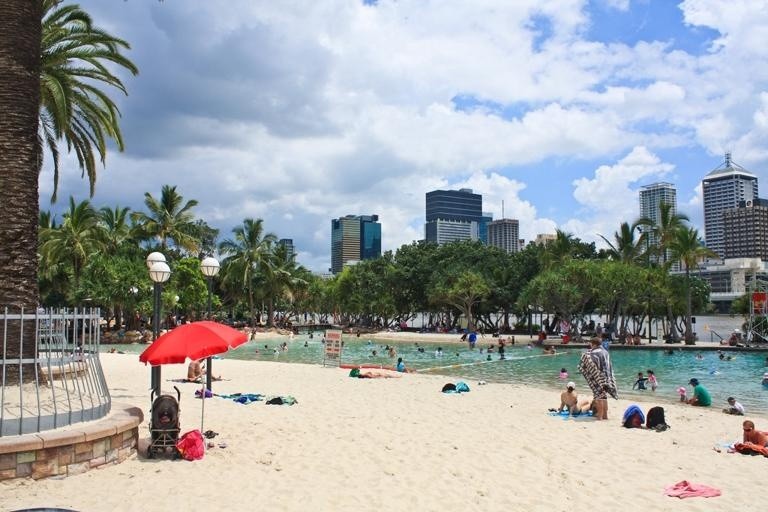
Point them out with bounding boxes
[147,384,182,460]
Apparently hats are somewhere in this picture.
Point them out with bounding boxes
[567,382,575,389]
[687,377,698,384]
[160,411,170,418]
[587,337,602,344]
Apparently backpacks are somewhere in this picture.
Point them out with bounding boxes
[646,407,670,432]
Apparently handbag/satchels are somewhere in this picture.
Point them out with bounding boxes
[176,429,205,461]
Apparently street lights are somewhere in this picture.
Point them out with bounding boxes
[200,257,221,394]
[147,253,173,443]
[527,303,534,337]
[537,304,544,334]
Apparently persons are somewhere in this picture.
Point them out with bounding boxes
[102,314,768,457]
